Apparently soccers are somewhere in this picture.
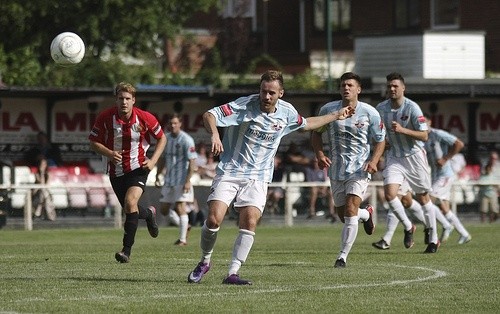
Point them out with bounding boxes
[50,32,86,66]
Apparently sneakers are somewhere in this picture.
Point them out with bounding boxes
[115,251,130,264]
[188,262,210,283]
[424,240,441,254]
[145,206,158,238]
[334,258,345,268]
[363,204,373,235]
[440,225,454,243]
[458,235,472,245]
[371,239,390,249]
[222,273,252,285]
[404,224,417,249]
[424,225,430,245]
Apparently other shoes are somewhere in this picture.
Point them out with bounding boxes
[174,239,187,246]
[187,225,192,231]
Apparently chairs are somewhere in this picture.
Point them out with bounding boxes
[12,166,117,218]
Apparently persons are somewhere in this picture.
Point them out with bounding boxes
[196,142,337,223]
[371,113,472,249]
[311,72,386,268]
[34,130,61,167]
[88,81,167,263]
[188,70,356,285]
[154,112,197,245]
[376,72,440,253]
[478,151,500,224]
